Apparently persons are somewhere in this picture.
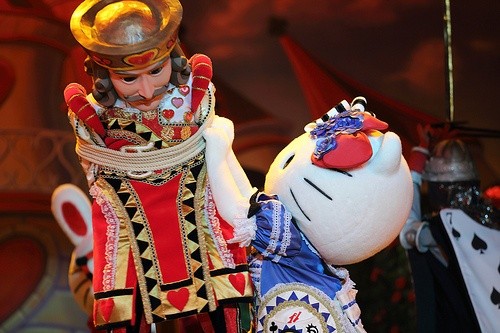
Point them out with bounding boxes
[64,1,266,332]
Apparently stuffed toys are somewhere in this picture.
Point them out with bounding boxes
[198,93,414,333]
